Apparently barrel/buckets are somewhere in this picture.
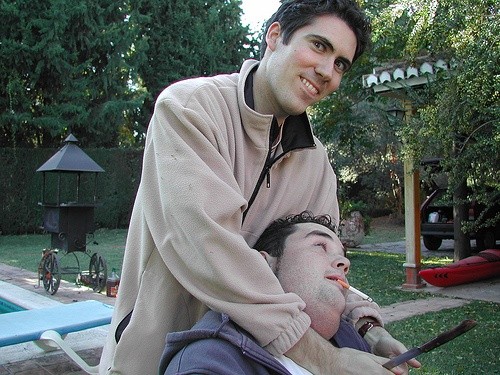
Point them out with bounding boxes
[428,212,439,223]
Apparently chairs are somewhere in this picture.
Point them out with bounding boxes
[0,299,116,375]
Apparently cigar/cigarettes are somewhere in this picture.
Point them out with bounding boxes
[336,279,373,303]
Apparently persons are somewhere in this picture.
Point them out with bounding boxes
[161,210,373,375]
[99,0,421,375]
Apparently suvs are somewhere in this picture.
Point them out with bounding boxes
[419,159,500,251]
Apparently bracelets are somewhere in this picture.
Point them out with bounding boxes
[357,321,383,338]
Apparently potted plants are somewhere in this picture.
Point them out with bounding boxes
[339,199,373,249]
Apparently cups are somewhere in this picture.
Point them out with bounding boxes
[438,210,442,222]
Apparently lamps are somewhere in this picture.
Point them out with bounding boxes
[386,103,410,131]
